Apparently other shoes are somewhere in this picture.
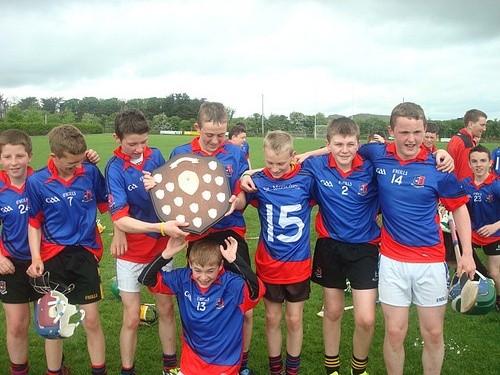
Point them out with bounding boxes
[495,304,500,313]
[162,368,180,375]
[238,366,255,375]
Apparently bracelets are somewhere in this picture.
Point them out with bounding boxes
[160,222,166,236]
[32,258,41,261]
[241,174,251,177]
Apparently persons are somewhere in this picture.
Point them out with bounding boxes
[26,125,127,375]
[142,102,253,375]
[239,131,316,375]
[422,123,438,153]
[294,102,476,375]
[459,146,500,310]
[105,109,190,375]
[446,109,500,182]
[240,117,455,375]
[0,130,64,375]
[368,132,390,144]
[228,123,252,170]
[138,232,266,375]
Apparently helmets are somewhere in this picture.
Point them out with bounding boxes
[34,290,85,340]
[110,276,120,299]
[449,270,497,315]
[95,219,107,234]
[139,303,156,325]
